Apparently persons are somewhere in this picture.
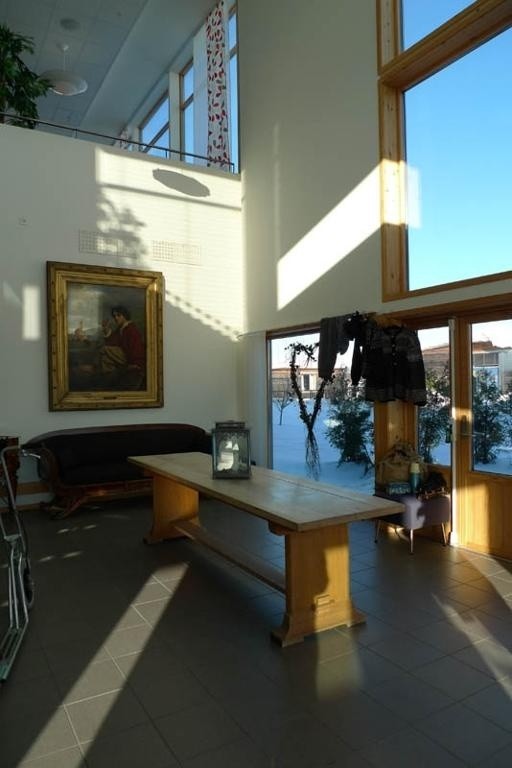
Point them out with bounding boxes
[78,303,146,391]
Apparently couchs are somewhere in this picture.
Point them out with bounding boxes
[23,424,212,519]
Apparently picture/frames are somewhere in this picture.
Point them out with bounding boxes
[46,260,163,411]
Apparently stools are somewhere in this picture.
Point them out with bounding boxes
[372,484,451,554]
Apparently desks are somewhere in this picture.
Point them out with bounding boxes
[130,447,408,647]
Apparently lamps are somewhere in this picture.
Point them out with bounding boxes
[39,43,89,98]
